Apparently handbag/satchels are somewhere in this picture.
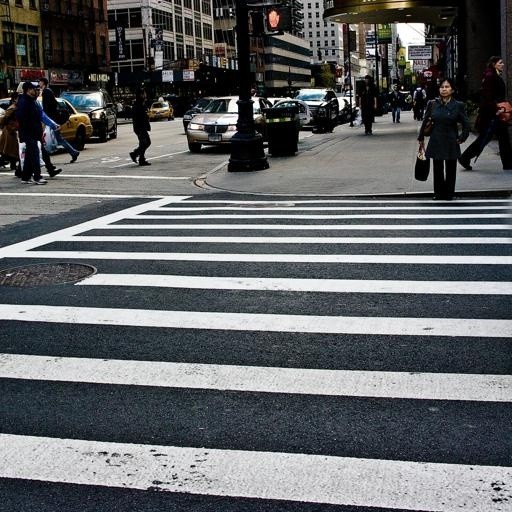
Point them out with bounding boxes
[415,145,429,182]
[423,100,434,137]
[58,109,69,125]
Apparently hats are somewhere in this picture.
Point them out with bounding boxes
[23,81,40,89]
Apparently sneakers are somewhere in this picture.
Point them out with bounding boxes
[21,179,35,184]
[34,178,48,185]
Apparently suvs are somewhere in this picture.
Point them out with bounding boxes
[57,87,118,144]
[291,88,339,134]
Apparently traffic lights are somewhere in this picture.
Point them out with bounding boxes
[199,59,203,73]
[265,4,292,33]
[316,50,322,61]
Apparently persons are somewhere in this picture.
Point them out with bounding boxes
[418,77,469,200]
[458,56,512,172]
[129,88,153,167]
[0,73,81,187]
[361,70,427,135]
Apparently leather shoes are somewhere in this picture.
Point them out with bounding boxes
[139,161,151,166]
[70,152,80,163]
[130,152,138,163]
[49,168,62,177]
[458,155,472,170]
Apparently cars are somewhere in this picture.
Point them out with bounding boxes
[1,82,93,152]
[337,99,354,125]
[148,96,174,123]
[266,97,291,108]
[337,96,359,118]
[264,96,296,110]
[186,96,310,151]
[183,97,219,134]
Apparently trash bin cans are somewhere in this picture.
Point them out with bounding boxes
[266,108,298,158]
